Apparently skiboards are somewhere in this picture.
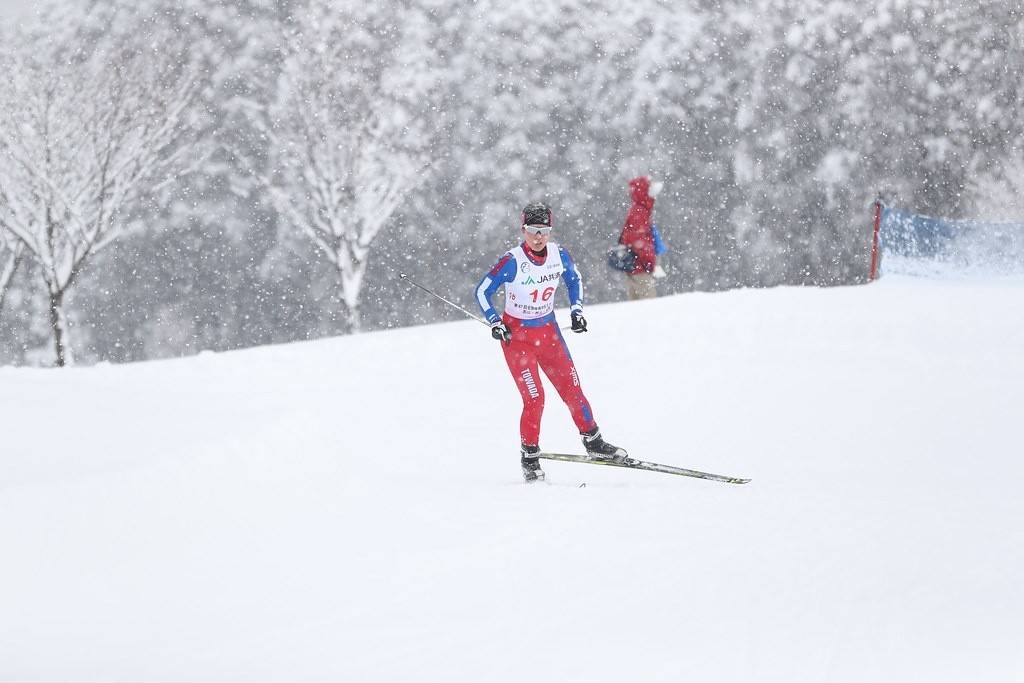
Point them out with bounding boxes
[523,452,753,489]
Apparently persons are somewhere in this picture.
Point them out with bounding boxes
[475,203,628,481]
[622,177,662,300]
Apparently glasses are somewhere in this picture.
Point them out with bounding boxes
[524,226,552,235]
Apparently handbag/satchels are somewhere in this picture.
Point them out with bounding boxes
[607,243,637,271]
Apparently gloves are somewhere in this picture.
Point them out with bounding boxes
[491,320,511,341]
[571,312,588,334]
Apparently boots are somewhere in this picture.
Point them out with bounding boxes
[580,426,628,464]
[521,444,545,482]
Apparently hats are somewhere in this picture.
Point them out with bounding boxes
[648,182,663,197]
[522,203,552,226]
